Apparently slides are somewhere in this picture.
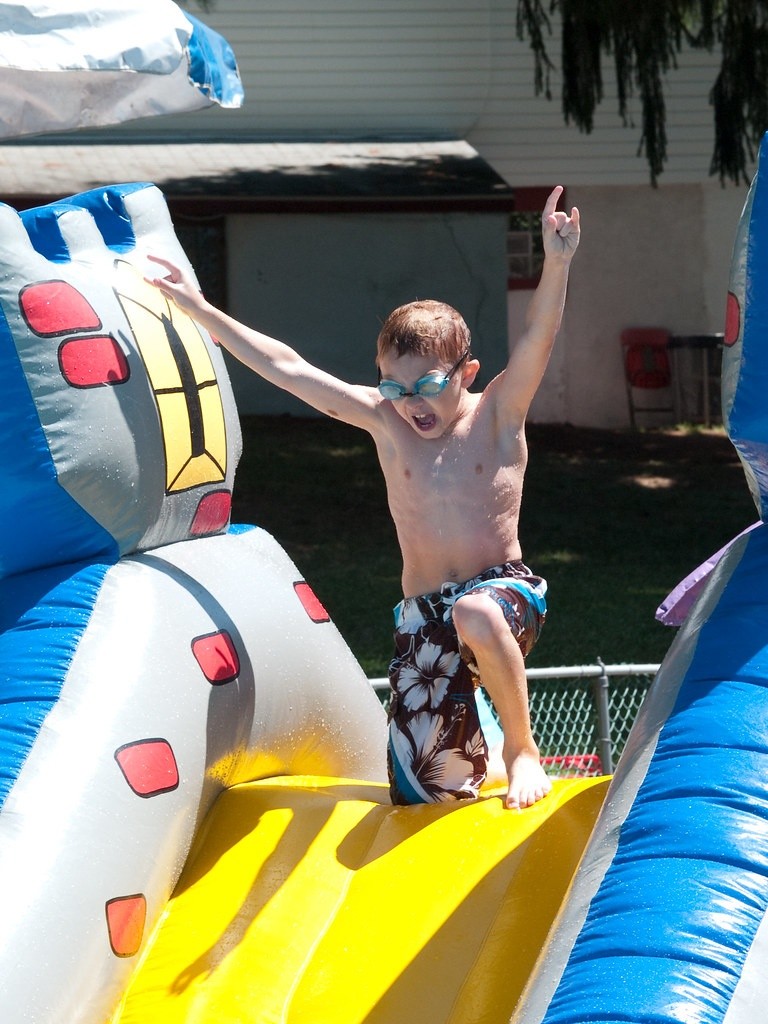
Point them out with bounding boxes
[0,132,768,1023]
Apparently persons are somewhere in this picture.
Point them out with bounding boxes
[143,184,580,809]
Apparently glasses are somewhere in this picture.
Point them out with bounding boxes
[379,348,470,403]
